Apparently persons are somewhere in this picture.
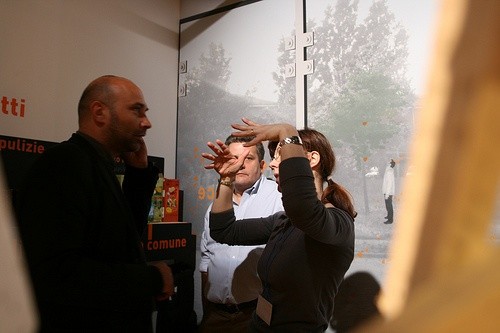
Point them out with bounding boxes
[199,135,285,333]
[6,75,174,333]
[202,116,355,333]
[382,159,396,225]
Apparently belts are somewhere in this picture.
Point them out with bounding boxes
[206,298,259,313]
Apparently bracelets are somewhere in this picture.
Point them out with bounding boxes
[219,179,235,187]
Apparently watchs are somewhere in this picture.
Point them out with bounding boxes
[279,136,303,147]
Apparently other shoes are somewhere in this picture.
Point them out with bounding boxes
[385,217,388,219]
[384,221,393,224]
